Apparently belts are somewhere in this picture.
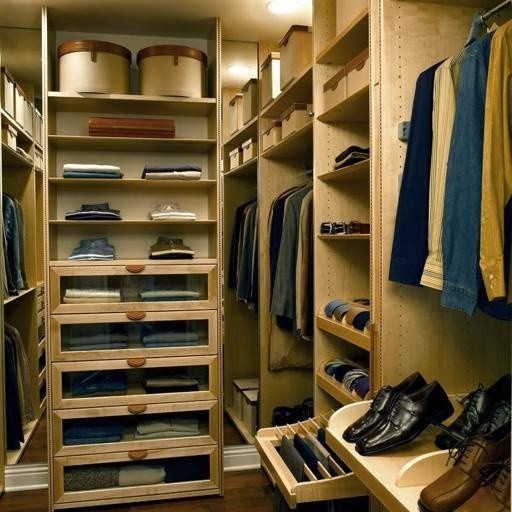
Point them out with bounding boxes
[321,221,370,234]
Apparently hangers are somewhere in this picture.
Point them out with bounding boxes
[475,5,504,37]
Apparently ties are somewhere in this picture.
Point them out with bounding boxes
[325,299,370,331]
[324,354,370,399]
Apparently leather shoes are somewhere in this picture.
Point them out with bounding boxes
[343,372,511,512]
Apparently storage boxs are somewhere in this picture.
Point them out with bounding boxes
[136,42,211,100]
[227,0,371,173]
[54,38,134,99]
[230,372,260,421]
[1,66,44,175]
[243,389,263,436]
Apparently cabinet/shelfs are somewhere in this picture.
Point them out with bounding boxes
[313,0,388,424]
[262,0,316,441]
[221,43,265,448]
[252,388,496,512]
[374,0,512,511]
[40,3,226,512]
[1,106,45,497]
